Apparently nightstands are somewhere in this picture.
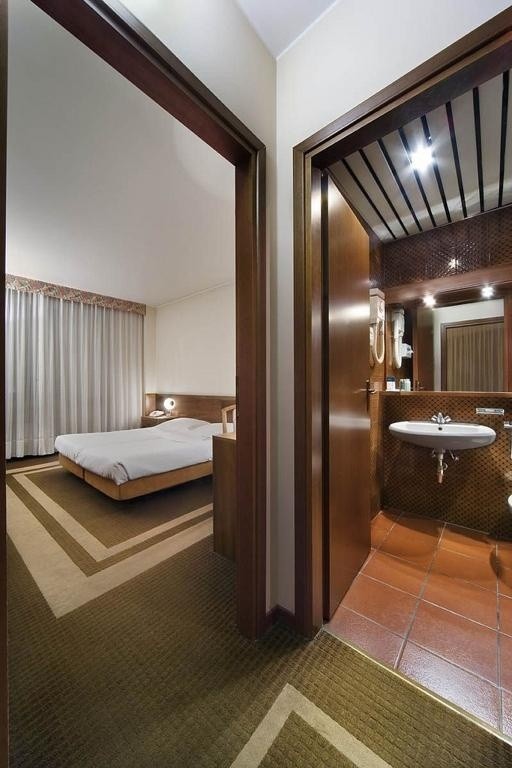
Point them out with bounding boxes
[141,416,177,427]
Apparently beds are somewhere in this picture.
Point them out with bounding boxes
[55,418,235,509]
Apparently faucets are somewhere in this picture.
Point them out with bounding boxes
[431,411,452,423]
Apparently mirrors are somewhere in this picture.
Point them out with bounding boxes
[431,294,508,393]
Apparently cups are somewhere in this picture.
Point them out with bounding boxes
[386,378,396,390]
[398,378,412,390]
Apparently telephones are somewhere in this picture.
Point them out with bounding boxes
[149,410,164,417]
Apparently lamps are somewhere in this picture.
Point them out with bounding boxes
[163,398,176,416]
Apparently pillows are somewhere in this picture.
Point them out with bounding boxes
[156,418,234,440]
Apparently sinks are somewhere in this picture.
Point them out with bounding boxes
[389,420,497,453]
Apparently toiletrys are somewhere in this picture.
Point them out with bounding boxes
[404,379,411,391]
